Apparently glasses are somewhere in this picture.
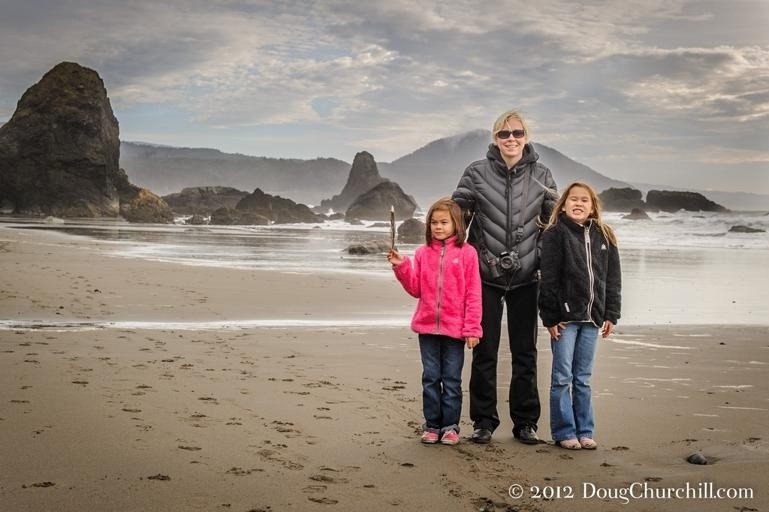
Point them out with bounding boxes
[495,129,525,139]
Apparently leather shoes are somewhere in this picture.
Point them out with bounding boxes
[518,424,541,445]
[470,428,492,444]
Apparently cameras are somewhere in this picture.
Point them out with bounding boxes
[488,251,521,281]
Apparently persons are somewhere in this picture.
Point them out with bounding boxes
[386,197,484,447]
[538,179,622,451]
[451,109,560,446]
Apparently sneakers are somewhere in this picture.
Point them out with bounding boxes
[559,436,597,450]
[440,429,459,446]
[421,431,439,444]
